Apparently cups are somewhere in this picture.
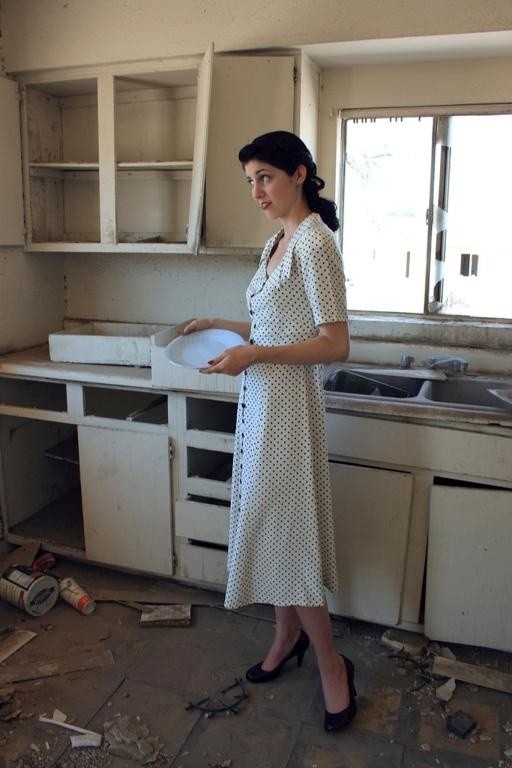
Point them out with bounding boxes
[59,577,96,616]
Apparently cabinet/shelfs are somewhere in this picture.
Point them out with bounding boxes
[2,369,177,582]
[0,43,216,256]
[319,409,511,654]
[201,47,320,257]
[178,391,243,594]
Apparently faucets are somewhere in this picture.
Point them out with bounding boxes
[423,354,468,372]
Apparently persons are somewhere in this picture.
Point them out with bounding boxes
[179,130,357,733]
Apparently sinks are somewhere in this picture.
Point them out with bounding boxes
[424,376,512,411]
[322,366,425,402]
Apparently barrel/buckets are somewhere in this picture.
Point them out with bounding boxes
[1,564,60,619]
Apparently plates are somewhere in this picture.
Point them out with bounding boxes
[163,329,248,369]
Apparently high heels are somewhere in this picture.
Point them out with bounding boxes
[246,629,310,683]
[324,652,358,732]
